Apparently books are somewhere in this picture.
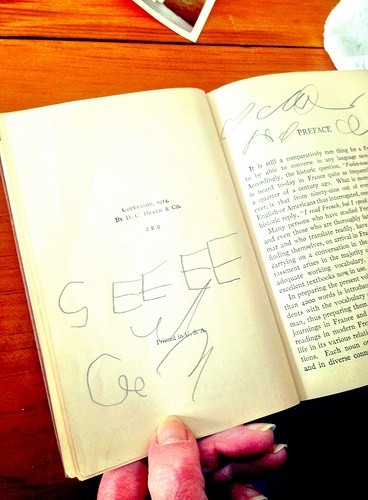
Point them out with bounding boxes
[2,71,368,482]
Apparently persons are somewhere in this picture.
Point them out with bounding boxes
[97,421,287,500]
